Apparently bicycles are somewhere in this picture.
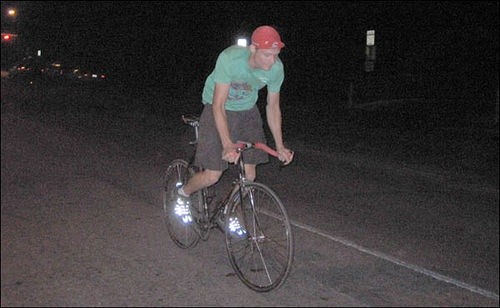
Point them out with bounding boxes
[157,114,295,293]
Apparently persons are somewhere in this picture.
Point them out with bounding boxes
[173,25,295,242]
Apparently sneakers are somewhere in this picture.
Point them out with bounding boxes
[172,182,194,226]
[224,211,248,239]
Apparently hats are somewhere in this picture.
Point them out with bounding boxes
[251,26,284,49]
